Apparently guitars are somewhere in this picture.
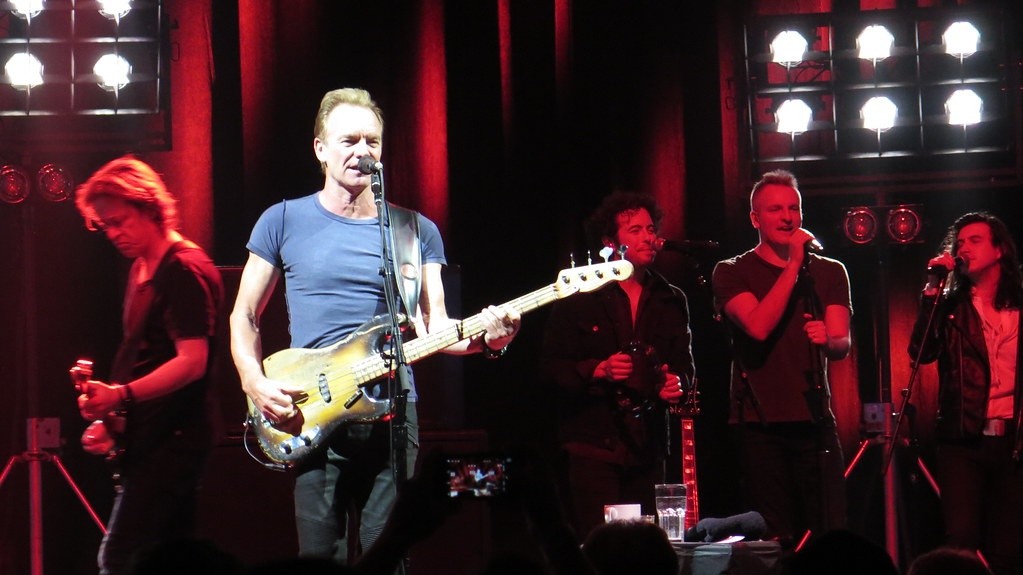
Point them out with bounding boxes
[669,377,701,533]
[245,242,634,469]
[71,361,131,496]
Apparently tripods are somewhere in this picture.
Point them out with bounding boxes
[794,400,991,571]
[0,414,107,575]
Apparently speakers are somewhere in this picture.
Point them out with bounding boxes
[193,264,495,575]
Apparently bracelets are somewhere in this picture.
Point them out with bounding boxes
[115,384,135,405]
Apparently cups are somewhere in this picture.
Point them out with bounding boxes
[605,503,640,524]
[655,483,685,542]
[640,517,655,525]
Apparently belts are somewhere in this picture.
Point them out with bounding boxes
[979,419,1018,436]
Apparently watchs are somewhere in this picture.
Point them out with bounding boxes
[480,330,510,360]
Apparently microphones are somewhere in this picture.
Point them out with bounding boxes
[357,155,384,174]
[654,238,720,253]
[928,255,968,275]
[805,239,823,253]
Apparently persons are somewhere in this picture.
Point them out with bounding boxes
[907,210,1022,550]
[578,522,999,575]
[121,532,351,575]
[75,153,225,575]
[229,86,523,572]
[711,168,854,529]
[563,191,697,522]
